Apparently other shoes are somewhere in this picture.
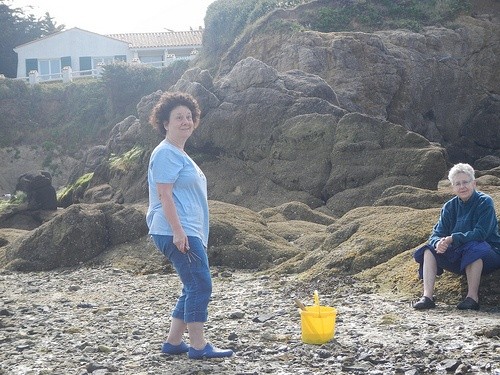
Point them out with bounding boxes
[457,297,480,310]
[161,341,190,354]
[413,296,435,309]
[189,343,233,358]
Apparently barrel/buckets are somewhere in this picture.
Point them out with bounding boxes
[301,289,337,345]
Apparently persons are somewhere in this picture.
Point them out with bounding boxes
[413,163,500,310]
[146,91,234,360]
